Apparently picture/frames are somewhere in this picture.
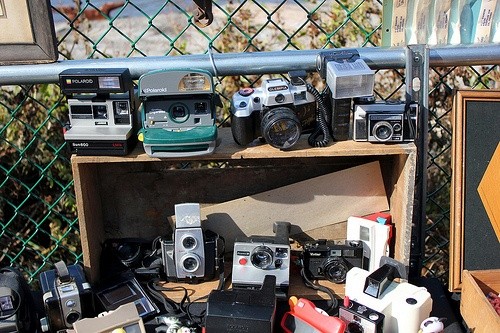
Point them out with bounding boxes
[447,89,500,295]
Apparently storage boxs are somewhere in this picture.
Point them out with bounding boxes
[458,267,500,333]
[67,127,421,306]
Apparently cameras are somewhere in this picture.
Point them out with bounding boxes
[136,66,222,159]
[351,100,419,143]
[339,297,384,333]
[161,202,221,283]
[233,237,291,289]
[229,77,319,150]
[300,241,364,283]
